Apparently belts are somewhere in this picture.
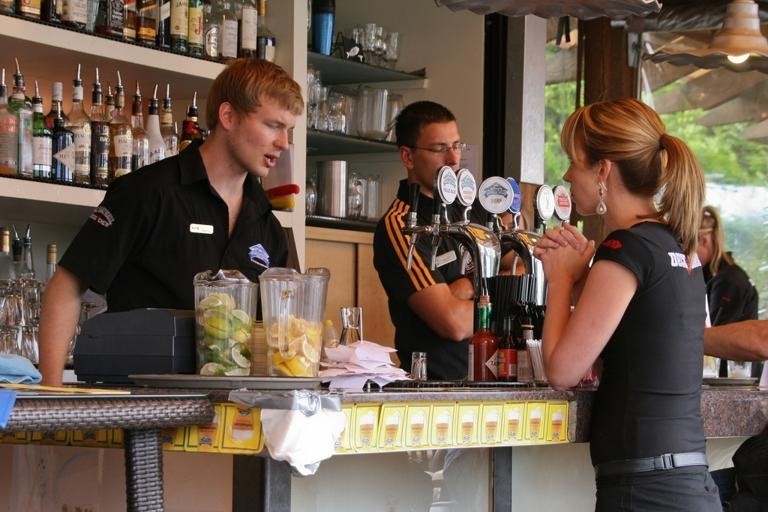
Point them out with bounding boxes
[594,452,709,479]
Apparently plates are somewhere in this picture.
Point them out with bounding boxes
[125,372,323,391]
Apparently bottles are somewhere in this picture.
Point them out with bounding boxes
[466,294,497,382]
[496,314,519,382]
[520,325,535,385]
[0,2,278,77]
[0,53,214,190]
[0,218,106,365]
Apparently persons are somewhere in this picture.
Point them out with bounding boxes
[373,101,476,382]
[534,99,724,511]
[703,320,768,509]
[38,58,304,389]
[690,206,759,379]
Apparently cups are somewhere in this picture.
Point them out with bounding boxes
[703,355,722,378]
[727,360,753,379]
[261,265,330,378]
[409,350,429,381]
[305,0,406,223]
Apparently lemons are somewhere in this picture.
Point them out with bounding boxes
[268,314,322,377]
[197,292,252,376]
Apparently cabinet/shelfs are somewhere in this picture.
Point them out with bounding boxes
[0,0,293,370]
[306,1,430,366]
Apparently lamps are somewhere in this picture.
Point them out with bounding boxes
[707,1,768,66]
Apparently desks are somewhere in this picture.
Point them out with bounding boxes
[2,392,214,512]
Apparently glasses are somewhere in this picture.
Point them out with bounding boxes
[407,141,468,153]
